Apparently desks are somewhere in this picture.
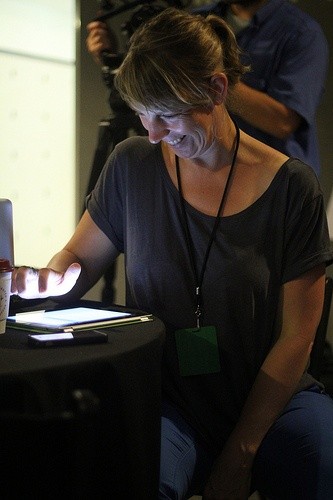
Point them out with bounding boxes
[0,294,167,500]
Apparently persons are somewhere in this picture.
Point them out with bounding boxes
[8,9,333,500]
[86,0,329,221]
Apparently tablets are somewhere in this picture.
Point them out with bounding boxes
[6,303,153,333]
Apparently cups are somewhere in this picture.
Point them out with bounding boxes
[0,259,14,334]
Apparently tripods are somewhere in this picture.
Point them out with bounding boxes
[83,112,149,302]
